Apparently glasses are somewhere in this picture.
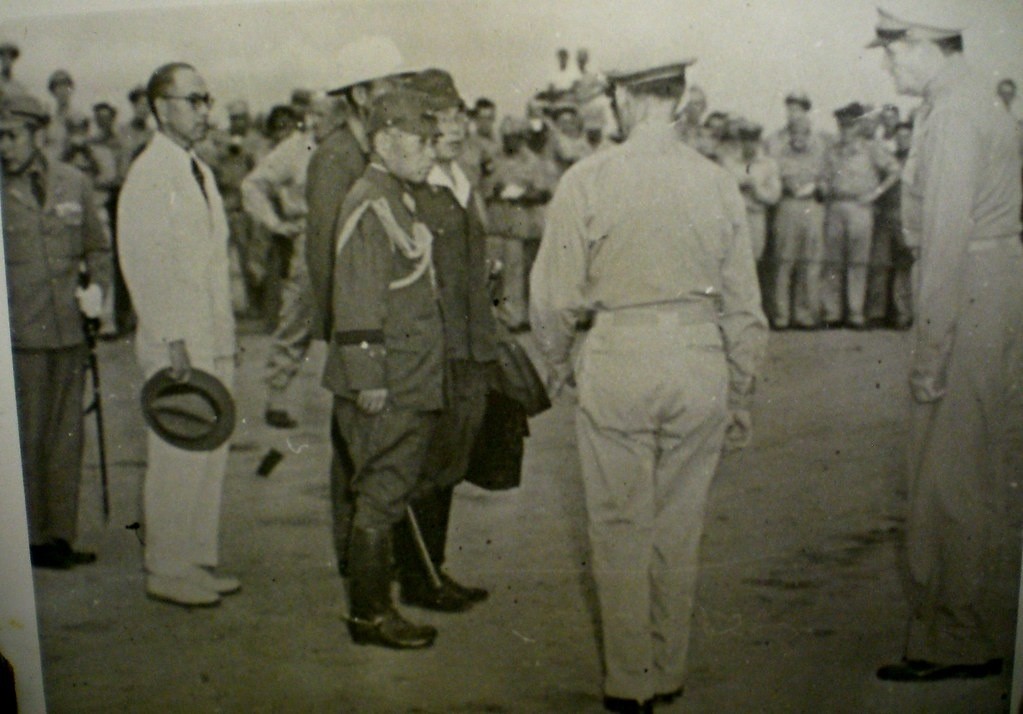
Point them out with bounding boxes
[162,94,214,110]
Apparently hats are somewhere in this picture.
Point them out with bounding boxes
[734,120,763,142]
[596,46,698,87]
[410,68,466,109]
[834,102,864,121]
[863,3,964,50]
[0,95,51,129]
[140,368,236,451]
[365,90,442,140]
[786,88,813,110]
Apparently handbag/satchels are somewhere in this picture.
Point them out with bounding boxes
[466,391,533,491]
[498,332,554,415]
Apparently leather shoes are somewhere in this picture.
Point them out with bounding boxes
[877,660,1000,682]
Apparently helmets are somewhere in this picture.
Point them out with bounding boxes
[324,36,420,96]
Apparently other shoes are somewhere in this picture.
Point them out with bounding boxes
[267,406,297,428]
[604,688,684,713]
[31,539,96,569]
[347,608,436,648]
[182,568,241,593]
[145,573,219,606]
[400,572,486,606]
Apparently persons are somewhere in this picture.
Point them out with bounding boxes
[320,89,449,649]
[116,62,246,604]
[0,2,1023,714]
[529,53,769,714]
